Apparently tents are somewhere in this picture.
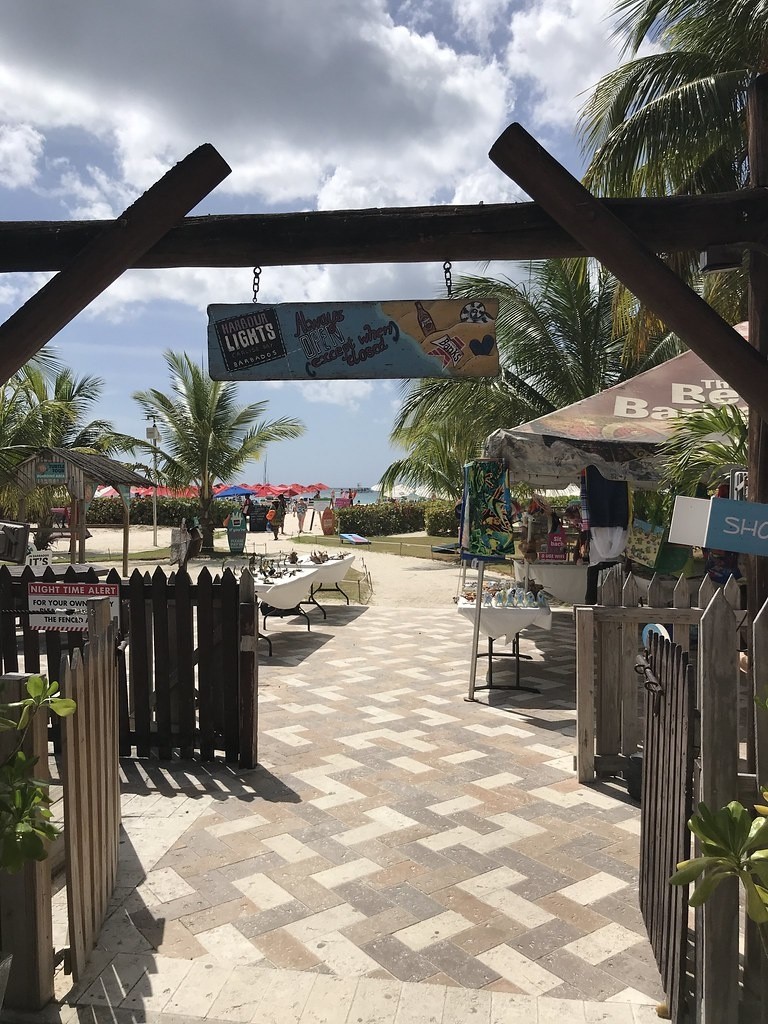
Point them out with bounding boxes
[457,320,759,709]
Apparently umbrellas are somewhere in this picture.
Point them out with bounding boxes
[370,479,469,501]
[94,479,329,501]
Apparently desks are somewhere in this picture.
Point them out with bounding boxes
[220,566,319,657]
[273,553,355,619]
[457,584,574,694]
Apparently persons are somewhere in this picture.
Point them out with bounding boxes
[293,497,308,533]
[572,530,591,565]
[274,492,287,533]
[240,492,254,532]
[313,490,322,515]
[340,487,358,508]
[267,498,285,542]
[329,488,336,510]
